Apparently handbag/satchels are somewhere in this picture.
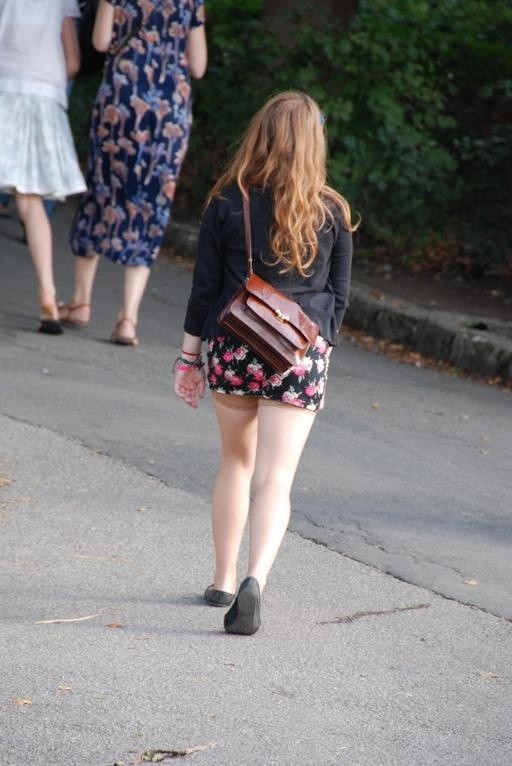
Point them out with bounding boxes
[217,266,320,374]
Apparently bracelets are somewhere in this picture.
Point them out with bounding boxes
[175,350,205,370]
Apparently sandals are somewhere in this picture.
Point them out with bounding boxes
[111,317,138,346]
[40,305,63,334]
[59,301,91,327]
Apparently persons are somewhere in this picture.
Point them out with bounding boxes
[58,1,208,346]
[172,90,353,636]
[0,0,87,335]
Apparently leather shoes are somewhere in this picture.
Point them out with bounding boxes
[205,581,236,606]
[224,577,264,635]
